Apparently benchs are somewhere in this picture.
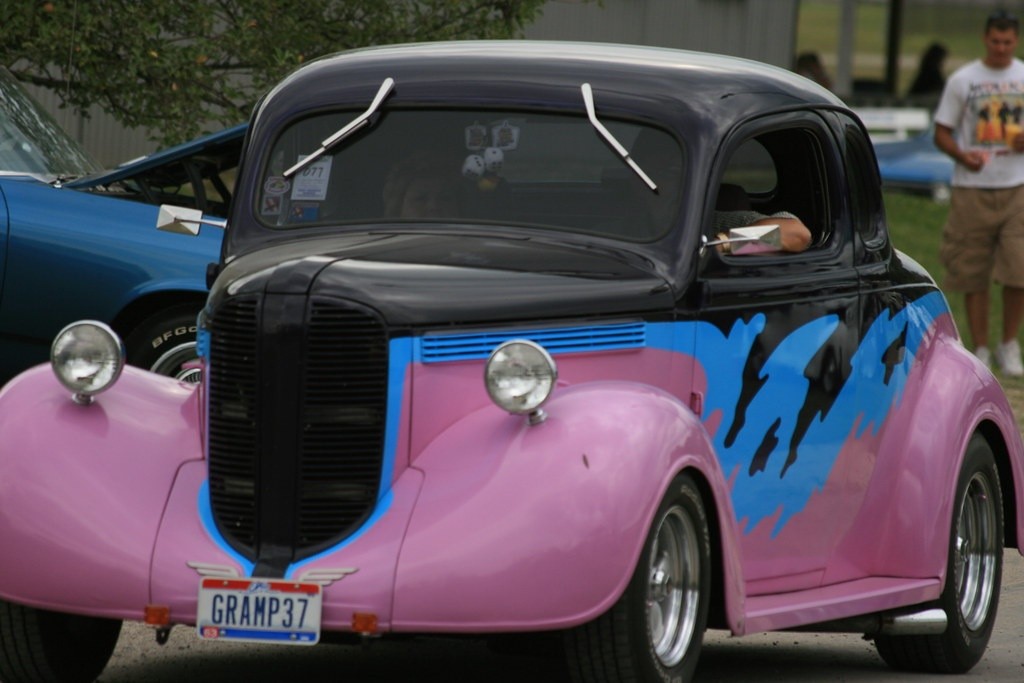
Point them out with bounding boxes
[852,105,950,201]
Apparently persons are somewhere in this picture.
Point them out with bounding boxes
[932,13,1024,380]
[906,43,949,105]
[795,51,831,87]
[623,125,814,258]
[381,153,465,219]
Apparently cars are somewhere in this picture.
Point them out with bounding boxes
[1,58,319,390]
[1,38,1024,683]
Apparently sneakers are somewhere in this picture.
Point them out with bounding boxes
[975,346,992,369]
[995,339,1024,378]
